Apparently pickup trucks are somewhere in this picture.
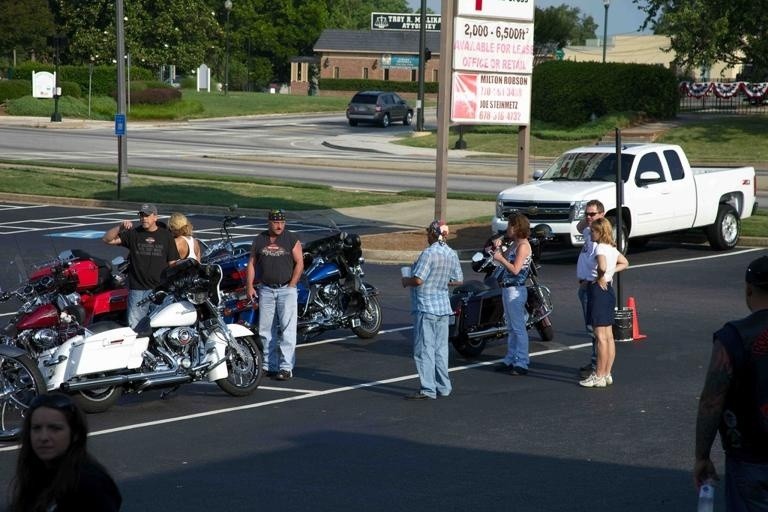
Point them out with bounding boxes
[493,141,760,252]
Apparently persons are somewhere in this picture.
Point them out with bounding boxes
[402,222,464,400]
[492,213,533,375]
[578,218,629,388]
[1,393,122,511]
[247,208,305,379]
[103,202,180,395]
[166,212,202,269]
[693,256,766,511]
[577,201,604,376]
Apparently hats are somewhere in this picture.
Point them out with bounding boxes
[137,202,158,216]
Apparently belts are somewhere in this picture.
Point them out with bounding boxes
[500,282,525,288]
[262,280,290,289]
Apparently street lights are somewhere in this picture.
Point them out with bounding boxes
[600,0,614,73]
[217,0,239,96]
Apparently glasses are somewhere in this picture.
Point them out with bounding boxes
[584,211,602,217]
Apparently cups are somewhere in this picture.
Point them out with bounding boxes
[400,266,412,279]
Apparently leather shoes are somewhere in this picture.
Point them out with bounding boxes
[405,390,434,400]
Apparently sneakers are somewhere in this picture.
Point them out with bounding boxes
[259,368,274,378]
[606,374,614,386]
[275,370,292,380]
[578,371,607,389]
[577,364,593,378]
[494,363,529,376]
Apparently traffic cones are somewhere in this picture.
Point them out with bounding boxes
[622,293,648,343]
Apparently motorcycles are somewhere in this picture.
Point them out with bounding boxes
[449,233,554,357]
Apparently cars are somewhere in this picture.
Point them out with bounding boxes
[345,87,415,129]
